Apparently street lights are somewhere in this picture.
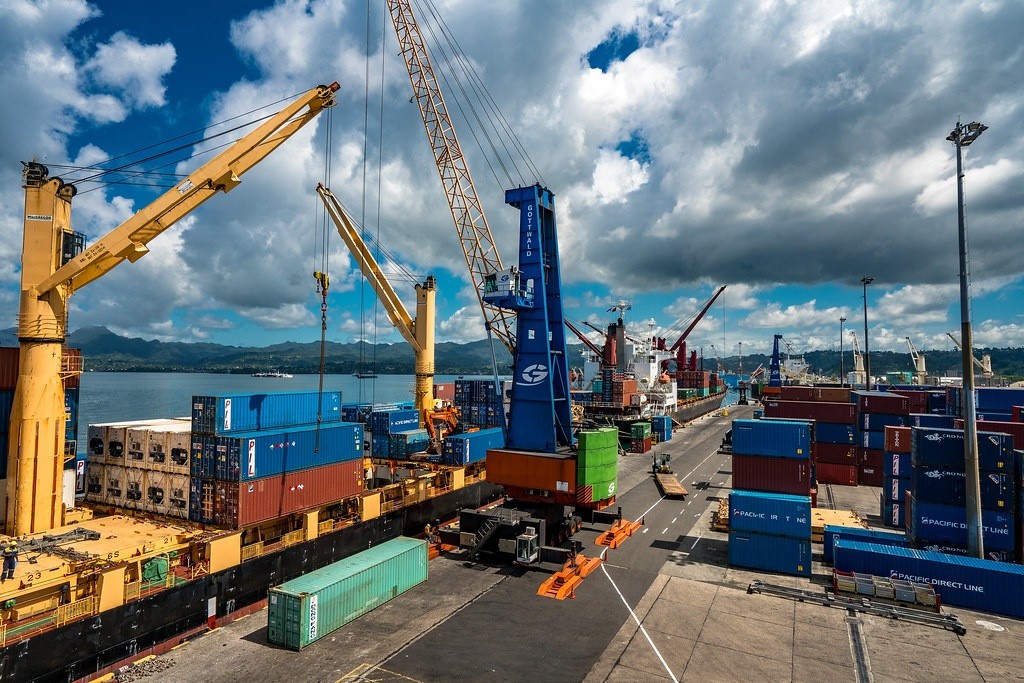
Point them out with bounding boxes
[860,273,875,392]
[839,317,847,388]
[738,341,743,380]
[945,115,989,560]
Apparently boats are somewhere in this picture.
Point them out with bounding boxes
[1,363,718,682]
[570,300,727,449]
[759,332,812,385]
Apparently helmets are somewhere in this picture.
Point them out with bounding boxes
[11,541,17,545]
[427,524,430,527]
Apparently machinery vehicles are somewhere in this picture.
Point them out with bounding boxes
[850,331,876,386]
[905,336,929,386]
[0,82,352,538]
[313,179,438,412]
[655,283,728,371]
[946,331,994,388]
[388,0,623,574]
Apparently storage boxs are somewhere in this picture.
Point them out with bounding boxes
[85,380,513,527]
[0,347,86,522]
[570,370,650,416]
[728,383,1024,617]
[630,416,672,453]
[266,536,429,653]
[676,371,724,400]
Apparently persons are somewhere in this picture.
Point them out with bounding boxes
[425,524,431,540]
[1,541,19,582]
